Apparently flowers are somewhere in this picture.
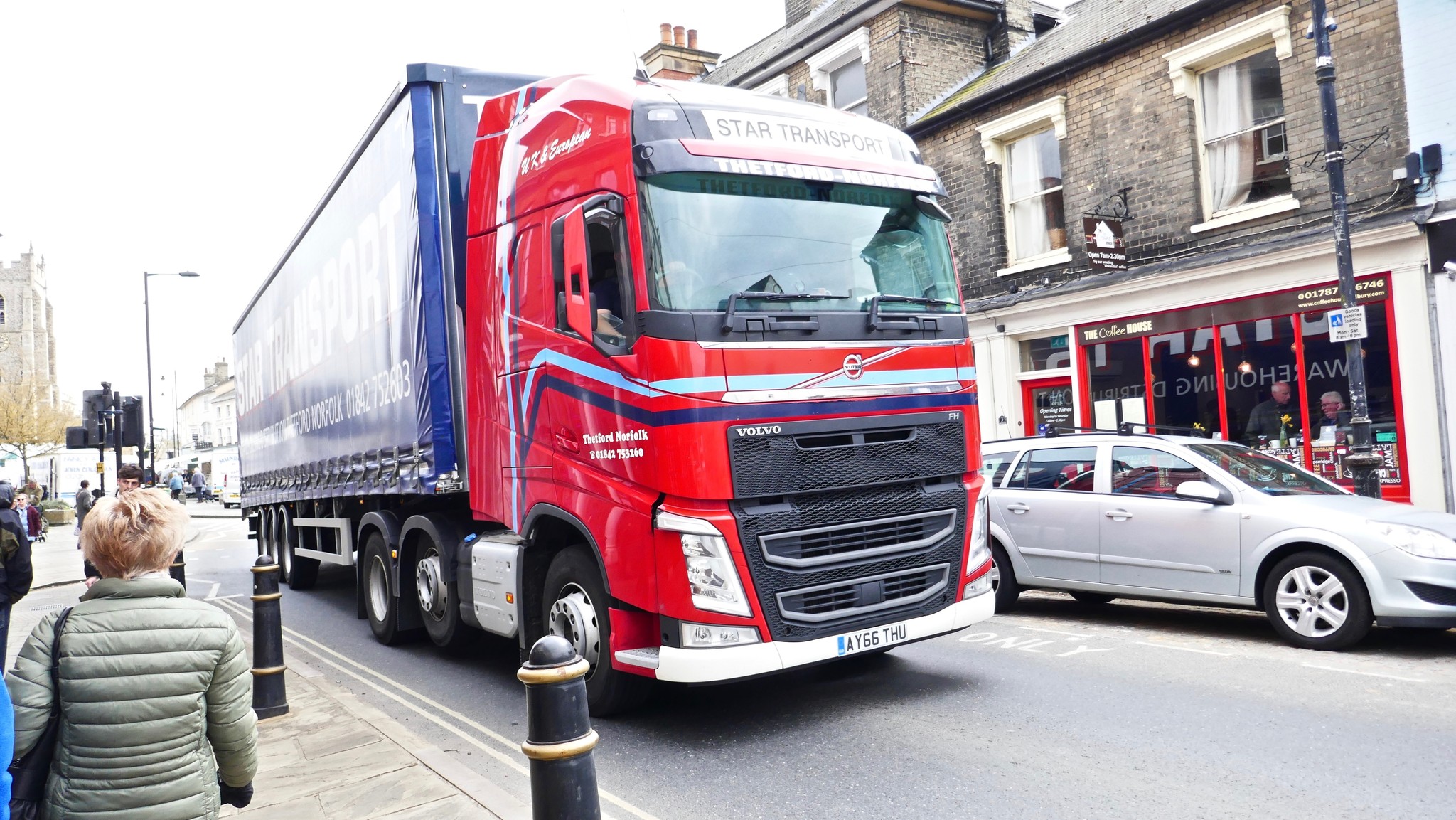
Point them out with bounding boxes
[1193,423,1206,431]
[1281,415,1293,428]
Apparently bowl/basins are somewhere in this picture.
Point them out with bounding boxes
[1310,441,1320,447]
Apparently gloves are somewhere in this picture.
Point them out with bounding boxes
[217,768,253,808]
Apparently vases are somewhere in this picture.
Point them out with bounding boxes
[1280,427,1289,448]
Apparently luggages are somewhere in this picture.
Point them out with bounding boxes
[203,488,214,503]
[178,493,186,505]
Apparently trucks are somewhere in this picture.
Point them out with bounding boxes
[211,452,240,497]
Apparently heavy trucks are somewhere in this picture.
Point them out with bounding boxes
[233,63,997,715]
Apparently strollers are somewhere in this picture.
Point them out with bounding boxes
[33,503,49,542]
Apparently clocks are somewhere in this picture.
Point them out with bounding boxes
[0,333,10,352]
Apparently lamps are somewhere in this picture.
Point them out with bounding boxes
[1041,276,1051,286]
[1443,261,1456,280]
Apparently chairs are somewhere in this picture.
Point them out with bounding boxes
[1165,464,1202,497]
[1127,465,1160,494]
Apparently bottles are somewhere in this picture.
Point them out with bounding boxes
[1296,429,1304,446]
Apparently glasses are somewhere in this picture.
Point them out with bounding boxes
[17,498,24,501]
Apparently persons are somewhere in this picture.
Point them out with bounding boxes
[182,470,191,498]
[1301,391,1352,446]
[13,493,42,555]
[6,487,258,820]
[1246,383,1302,450]
[11,479,44,516]
[170,472,184,500]
[84,463,144,589]
[0,485,34,670]
[0,669,14,820]
[191,467,206,503]
[76,480,93,549]
[595,240,687,332]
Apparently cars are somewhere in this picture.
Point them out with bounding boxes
[981,422,1456,650]
[223,475,241,509]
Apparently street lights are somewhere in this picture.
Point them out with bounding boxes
[144,271,200,486]
[161,370,180,457]
[161,388,175,458]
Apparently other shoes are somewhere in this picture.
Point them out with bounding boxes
[197,500,204,503]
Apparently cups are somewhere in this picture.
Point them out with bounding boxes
[1346,434,1353,444]
[811,288,831,295]
[1269,440,1280,449]
[1289,438,1296,448]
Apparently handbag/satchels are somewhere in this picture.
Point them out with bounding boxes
[74,526,80,536]
[8,606,74,820]
